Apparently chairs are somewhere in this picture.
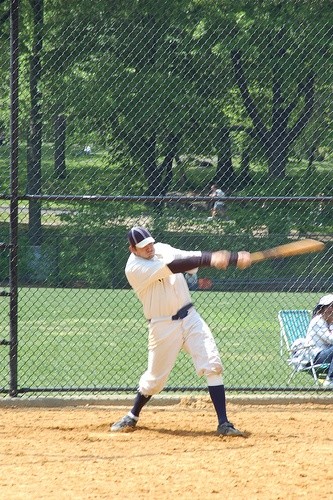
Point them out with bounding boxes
[278,310,333,388]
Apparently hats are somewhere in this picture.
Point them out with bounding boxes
[318,293,333,305]
[128,226,155,248]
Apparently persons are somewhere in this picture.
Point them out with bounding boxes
[110,227,253,437]
[206,185,227,221]
[298,293,333,391]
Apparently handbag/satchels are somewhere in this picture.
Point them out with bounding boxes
[292,338,312,366]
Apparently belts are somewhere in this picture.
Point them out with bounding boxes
[148,303,193,322]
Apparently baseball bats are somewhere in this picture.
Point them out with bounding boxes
[214,238,325,270]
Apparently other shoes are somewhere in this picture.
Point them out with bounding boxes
[323,381,333,387]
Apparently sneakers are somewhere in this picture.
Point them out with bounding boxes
[217,422,244,437]
[110,410,140,431]
